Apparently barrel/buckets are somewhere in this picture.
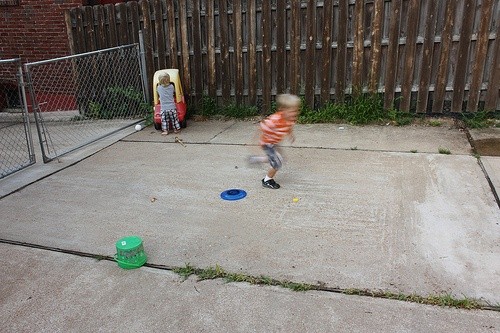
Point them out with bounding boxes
[114,238,147,269]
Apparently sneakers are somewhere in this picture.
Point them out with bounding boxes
[262,178,280,189]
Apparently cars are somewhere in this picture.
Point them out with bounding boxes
[153,69,188,130]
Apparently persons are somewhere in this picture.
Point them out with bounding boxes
[156,71,181,135]
[249,94,300,190]
